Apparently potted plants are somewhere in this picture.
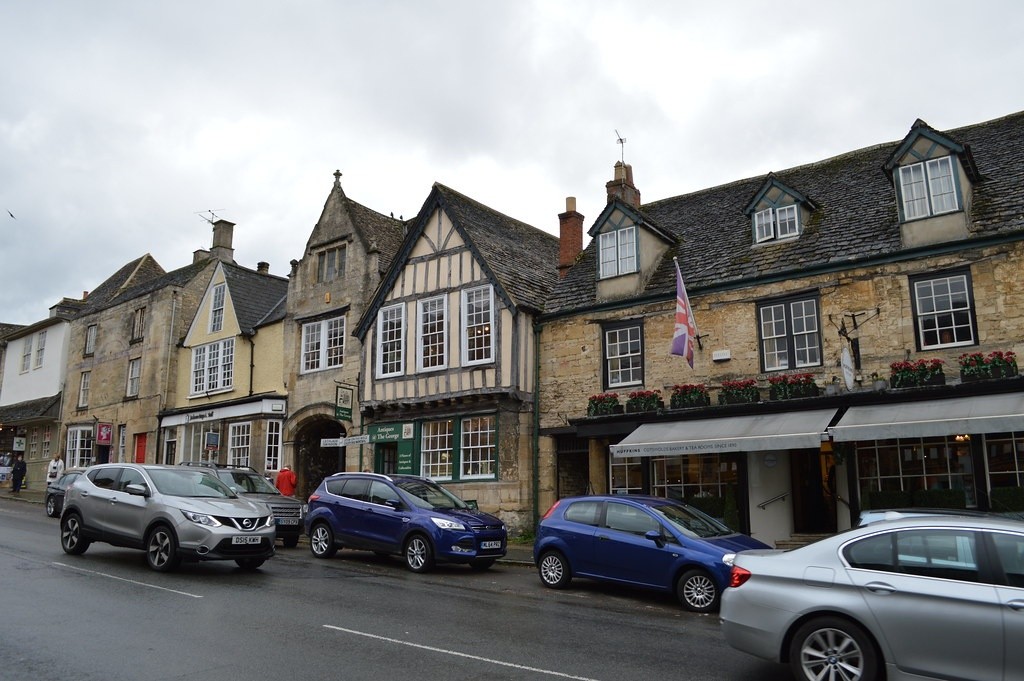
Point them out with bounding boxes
[871,376,888,391]
[823,380,841,395]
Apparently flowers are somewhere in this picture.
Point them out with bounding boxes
[768,373,815,401]
[890,357,944,388]
[587,390,619,416]
[717,378,758,406]
[625,389,663,412]
[670,384,708,406]
[958,350,1017,378]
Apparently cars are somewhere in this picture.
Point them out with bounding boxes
[718,514,1024,680]
[45,468,88,516]
[533,494,774,613]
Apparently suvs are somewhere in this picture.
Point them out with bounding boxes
[301,471,509,572]
[179,461,307,549]
[58,462,277,570]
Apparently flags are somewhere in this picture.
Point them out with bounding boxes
[670,262,696,371]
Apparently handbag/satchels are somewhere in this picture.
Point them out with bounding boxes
[49,472,57,477]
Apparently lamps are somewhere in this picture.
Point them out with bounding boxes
[954,433,971,443]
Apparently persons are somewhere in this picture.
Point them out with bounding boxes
[46,453,64,486]
[8,454,26,494]
[274,464,297,498]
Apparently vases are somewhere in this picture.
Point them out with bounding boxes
[769,383,820,401]
[718,392,761,405]
[586,404,624,417]
[626,401,665,413]
[670,397,711,409]
[960,364,1019,383]
[890,372,946,390]
